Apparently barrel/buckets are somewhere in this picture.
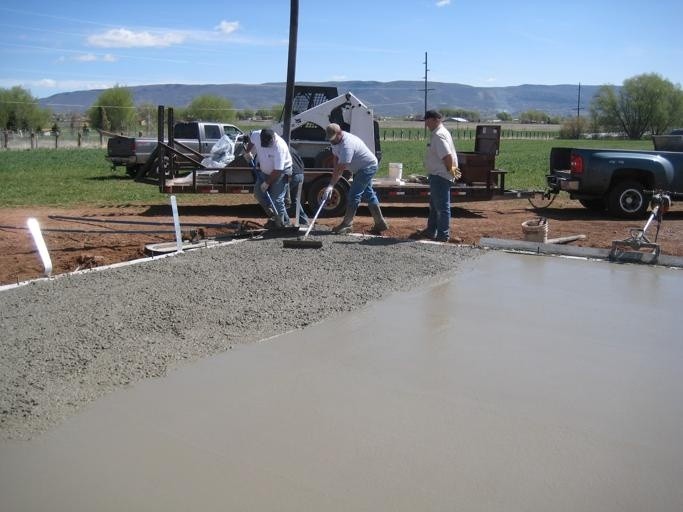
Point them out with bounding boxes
[519,218,548,244]
[388,162,403,181]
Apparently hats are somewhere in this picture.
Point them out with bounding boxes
[259,127,275,147]
[324,121,341,142]
[422,109,441,121]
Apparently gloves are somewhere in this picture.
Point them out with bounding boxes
[259,181,271,193]
[321,184,333,200]
[450,166,463,182]
[243,151,255,163]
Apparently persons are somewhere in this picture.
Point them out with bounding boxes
[283,142,309,225]
[321,122,390,234]
[241,127,292,227]
[415,108,462,242]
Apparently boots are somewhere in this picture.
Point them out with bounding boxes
[331,205,389,234]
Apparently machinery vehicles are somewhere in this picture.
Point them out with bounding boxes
[188,83,385,183]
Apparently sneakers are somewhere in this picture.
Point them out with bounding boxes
[416,227,449,243]
[263,217,311,230]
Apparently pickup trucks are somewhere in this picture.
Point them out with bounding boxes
[104,121,244,180]
[545,127,683,219]
